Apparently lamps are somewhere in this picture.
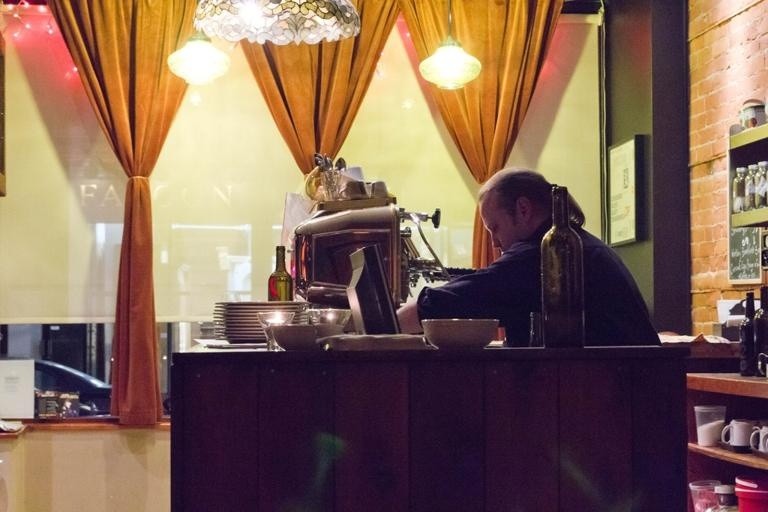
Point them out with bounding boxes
[166,1,483,93]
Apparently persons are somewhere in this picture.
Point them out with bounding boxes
[396,167,661,347]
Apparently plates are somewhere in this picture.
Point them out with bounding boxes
[212,299,310,347]
[317,334,425,350]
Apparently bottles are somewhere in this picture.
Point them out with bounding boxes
[539,185,589,348]
[268,244,294,301]
[731,161,768,214]
[739,284,768,379]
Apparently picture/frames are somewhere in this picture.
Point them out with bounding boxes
[608,134,641,247]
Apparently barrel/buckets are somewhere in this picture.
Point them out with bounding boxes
[734,475,768,512]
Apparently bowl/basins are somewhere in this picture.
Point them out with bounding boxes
[270,323,345,353]
[419,318,499,350]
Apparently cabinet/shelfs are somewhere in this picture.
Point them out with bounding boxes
[729,123,768,227]
[687,372,766,512]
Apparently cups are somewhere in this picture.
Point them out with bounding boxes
[257,311,297,353]
[693,406,768,451]
[306,307,353,334]
[688,477,734,512]
